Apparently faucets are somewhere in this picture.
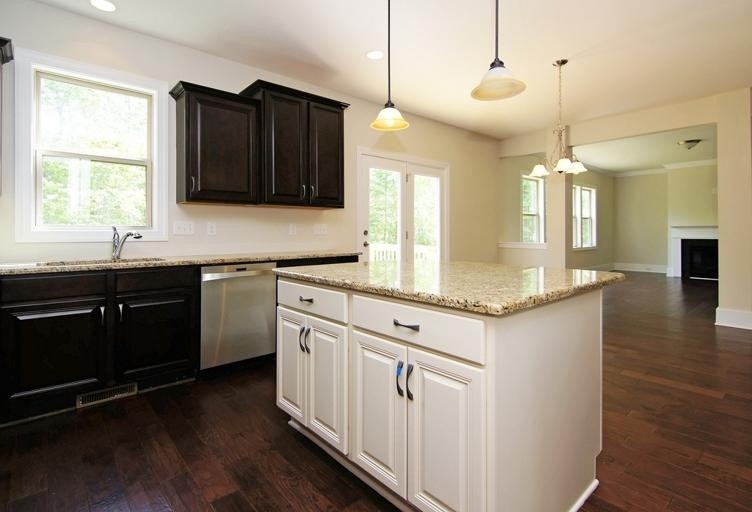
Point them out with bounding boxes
[112,227,143,260]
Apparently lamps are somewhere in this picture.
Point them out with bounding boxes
[471,0,528,105]
[525,57,589,182]
[368,0,412,139]
[678,137,701,150]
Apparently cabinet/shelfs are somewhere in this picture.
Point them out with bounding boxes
[1,266,209,429]
[270,275,348,468]
[240,78,353,210]
[347,288,611,512]
[168,78,260,206]
[281,254,361,269]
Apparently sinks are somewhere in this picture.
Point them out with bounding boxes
[36,258,165,266]
